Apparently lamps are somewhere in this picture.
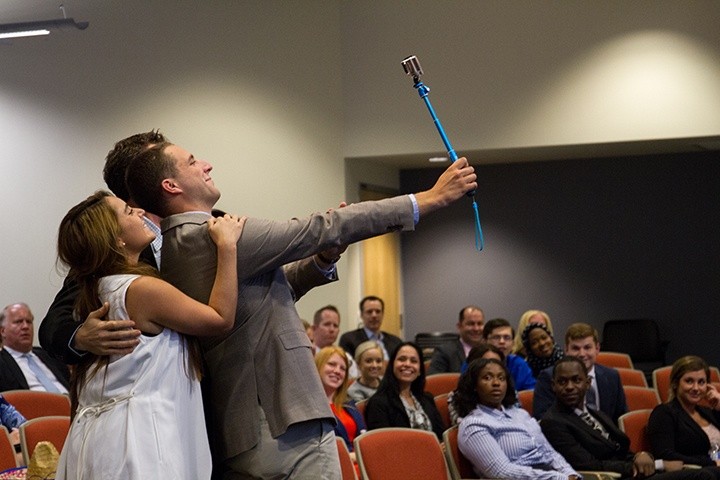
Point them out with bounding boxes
[0,4,90,40]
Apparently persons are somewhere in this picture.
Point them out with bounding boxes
[299,296,720,480]
[0,302,73,410]
[54,191,247,480]
[39,127,354,429]
[105,127,477,480]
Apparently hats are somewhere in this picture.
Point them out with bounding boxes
[522,323,554,357]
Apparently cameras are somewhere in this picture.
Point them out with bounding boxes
[400,55,425,78]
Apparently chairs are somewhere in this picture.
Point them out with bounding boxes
[336,317,720,480]
[0,390,73,473]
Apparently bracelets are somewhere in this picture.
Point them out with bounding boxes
[408,192,420,226]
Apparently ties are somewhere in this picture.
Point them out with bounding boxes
[21,354,58,393]
[586,387,596,410]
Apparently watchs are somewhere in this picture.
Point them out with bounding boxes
[317,250,341,265]
